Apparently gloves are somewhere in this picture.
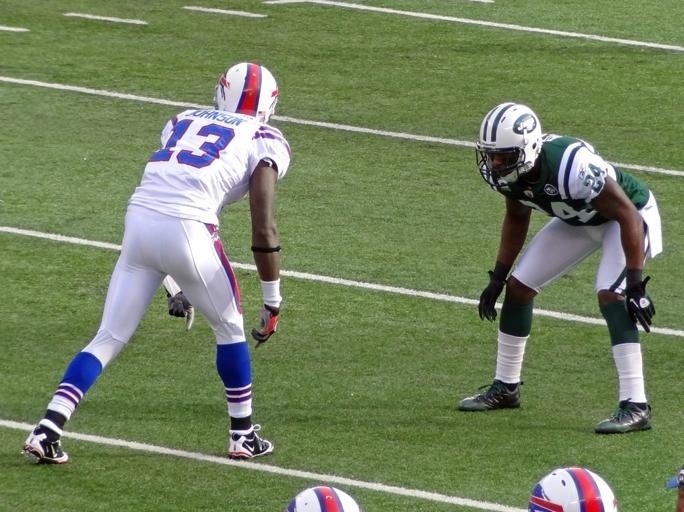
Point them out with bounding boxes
[251,304,280,348]
[478,271,507,323]
[626,276,655,333]
[167,291,194,332]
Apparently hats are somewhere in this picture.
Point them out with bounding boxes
[665,464,684,488]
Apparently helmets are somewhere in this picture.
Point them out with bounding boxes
[476,102,542,192]
[214,63,278,123]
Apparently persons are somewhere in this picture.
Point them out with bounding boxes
[677,465,684,512]
[22,62,292,464]
[459,104,664,434]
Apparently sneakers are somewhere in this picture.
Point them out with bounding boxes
[229,431,274,459]
[458,380,523,411]
[594,401,651,433]
[22,427,68,464]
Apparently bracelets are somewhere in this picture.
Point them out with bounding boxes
[259,279,283,308]
